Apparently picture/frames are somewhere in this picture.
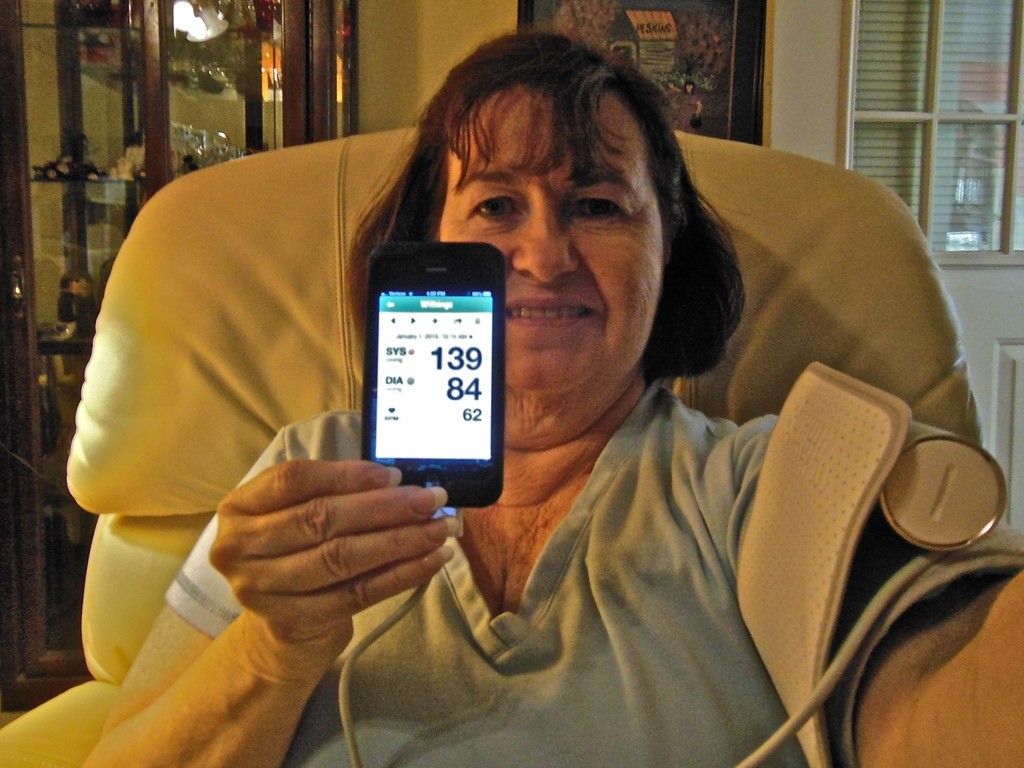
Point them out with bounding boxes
[518,0,767,146]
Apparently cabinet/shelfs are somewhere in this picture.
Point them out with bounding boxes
[0,0,358,717]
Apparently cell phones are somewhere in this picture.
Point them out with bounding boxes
[360,242,506,508]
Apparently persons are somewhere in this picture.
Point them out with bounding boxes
[79,29,1024,768]
[55,270,85,323]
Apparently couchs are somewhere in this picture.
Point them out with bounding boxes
[0,124,1024,768]
